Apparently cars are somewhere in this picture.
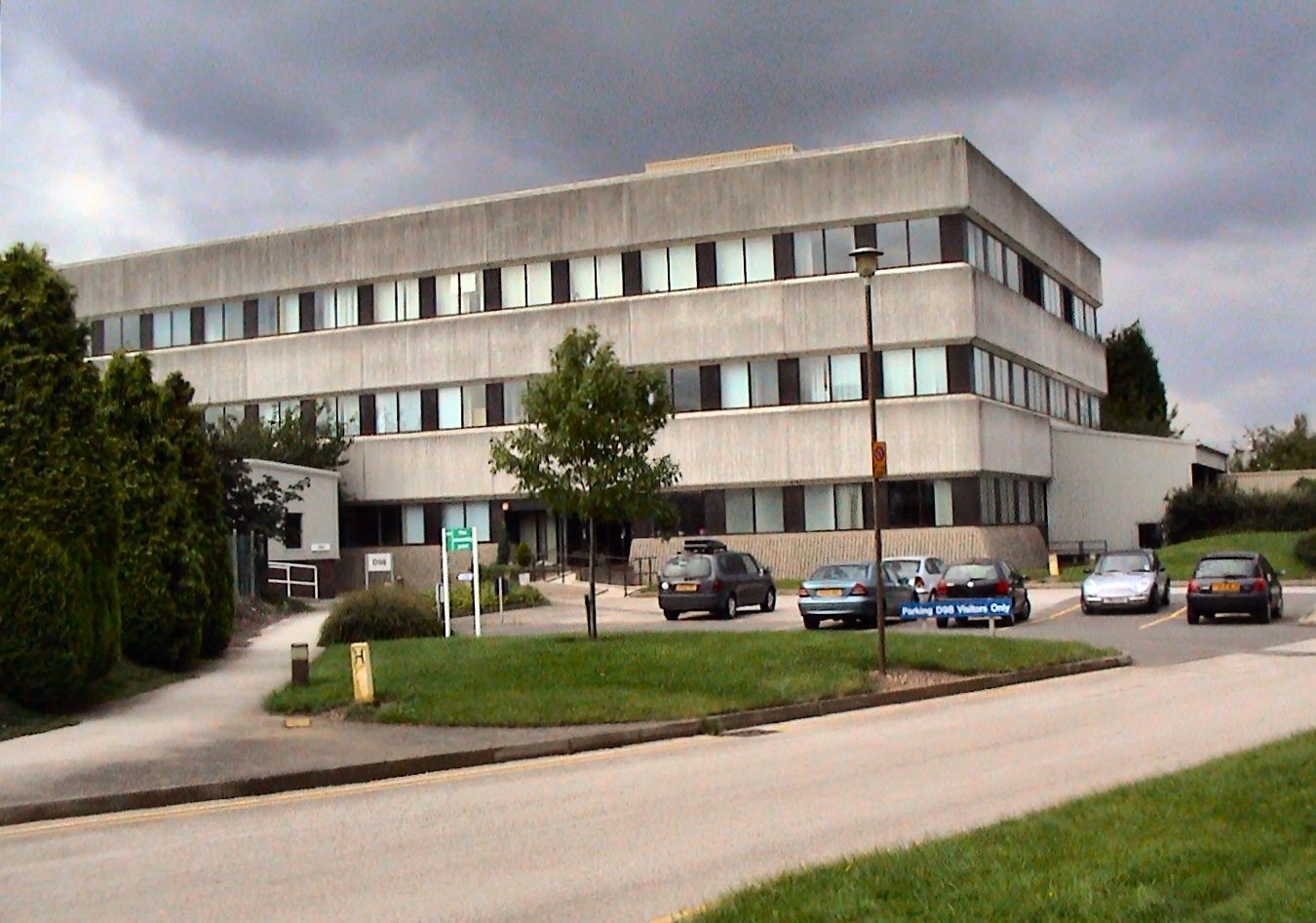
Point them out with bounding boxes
[934,559,1032,629]
[1186,552,1285,624]
[1079,549,1171,614]
[799,556,948,629]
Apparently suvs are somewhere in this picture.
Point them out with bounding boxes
[658,540,776,620]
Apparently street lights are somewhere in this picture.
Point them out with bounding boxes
[847,247,887,676]
